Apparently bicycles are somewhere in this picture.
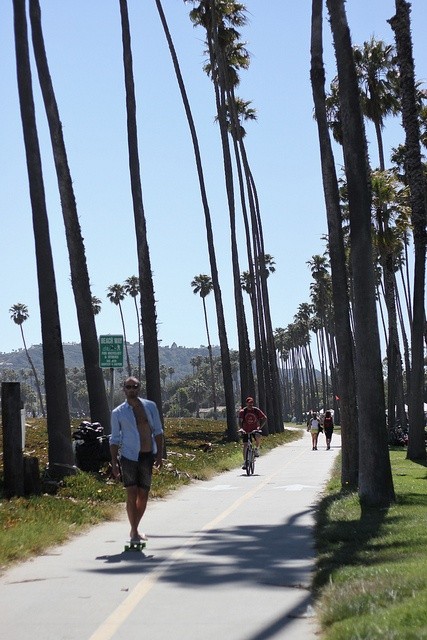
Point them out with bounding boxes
[236,429,261,475]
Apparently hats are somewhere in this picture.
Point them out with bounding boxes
[246,397,254,403]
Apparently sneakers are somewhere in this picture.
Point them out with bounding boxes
[255,448,260,456]
[313,446,314,450]
[315,446,317,450]
[242,462,249,469]
[327,445,330,450]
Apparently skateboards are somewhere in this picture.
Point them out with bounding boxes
[125,533,146,551]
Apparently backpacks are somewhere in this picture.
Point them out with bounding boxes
[325,417,333,431]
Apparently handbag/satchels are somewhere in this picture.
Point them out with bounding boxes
[318,424,323,432]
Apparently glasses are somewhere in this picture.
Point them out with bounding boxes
[123,383,139,389]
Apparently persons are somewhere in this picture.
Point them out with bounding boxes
[324,412,334,450]
[307,412,320,450]
[110,377,164,541]
[239,397,268,470]
